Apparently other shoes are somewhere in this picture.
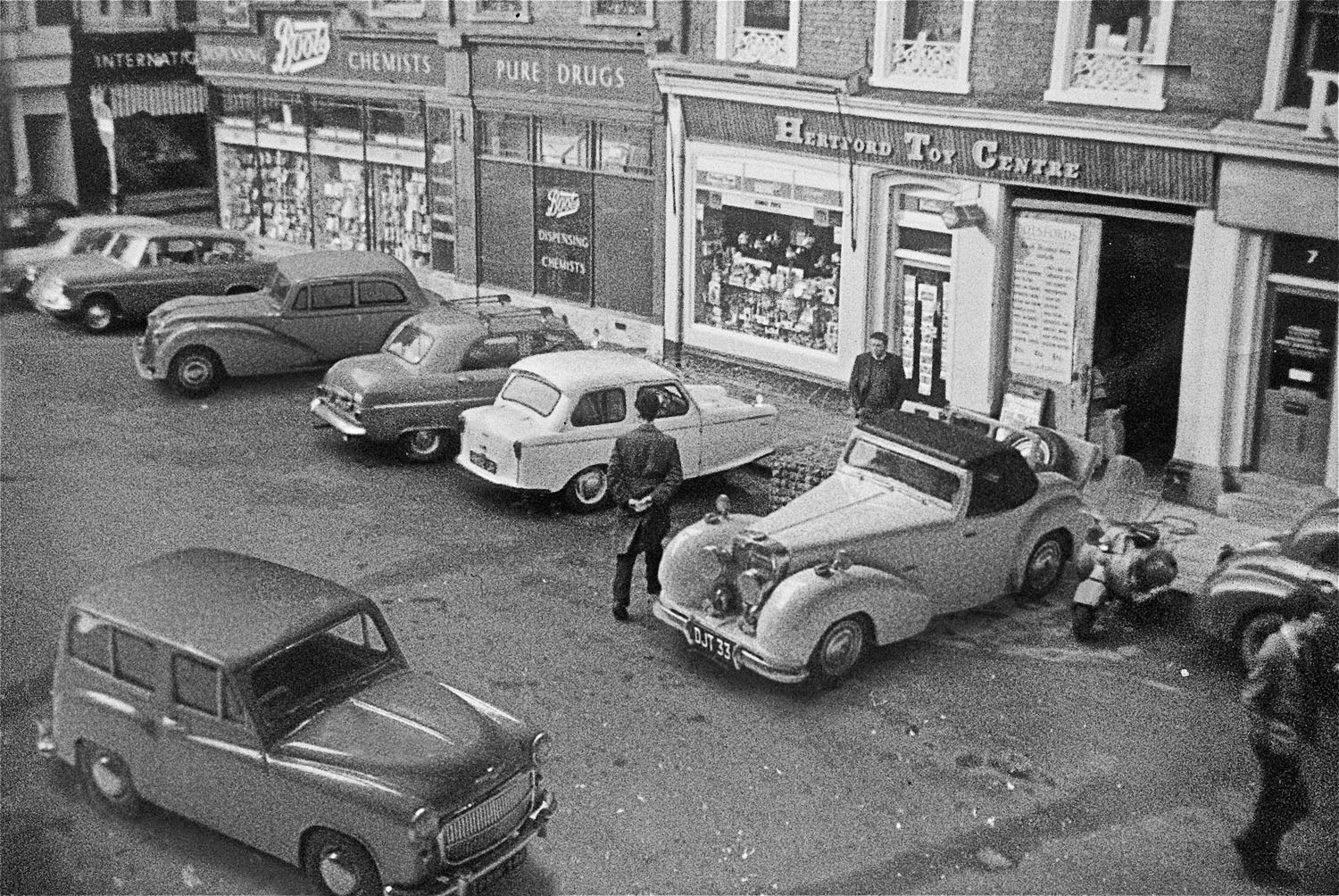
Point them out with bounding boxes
[1253,869,1297,888]
[1232,836,1257,874]
[612,602,629,620]
[647,583,662,596]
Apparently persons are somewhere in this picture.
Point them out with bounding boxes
[1233,589,1323,883]
[850,332,909,421]
[608,390,684,619]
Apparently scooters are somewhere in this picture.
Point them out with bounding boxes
[1069,456,1179,642]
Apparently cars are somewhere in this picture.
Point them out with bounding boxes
[649,411,1103,687]
[454,351,778,514]
[0,214,173,295]
[1195,498,1338,665]
[27,227,281,334]
[50,546,559,896]
[308,292,583,463]
[133,249,451,398]
